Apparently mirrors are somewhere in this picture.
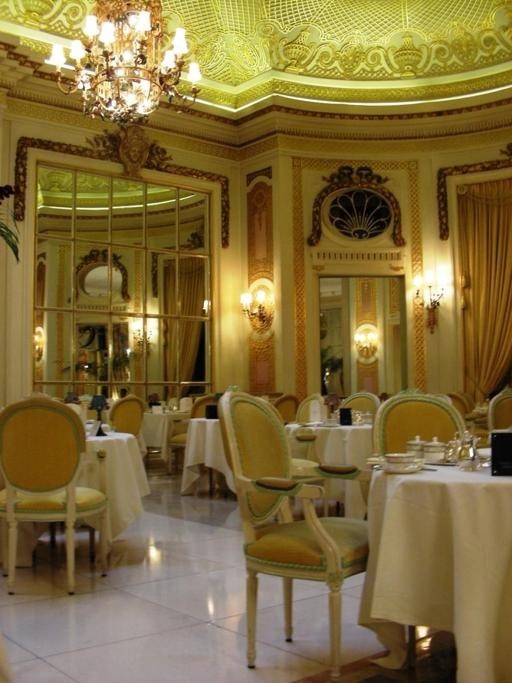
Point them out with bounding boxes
[11,139,230,406]
[319,270,407,409]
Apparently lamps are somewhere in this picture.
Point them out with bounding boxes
[414,270,446,311]
[240,290,266,324]
[45,0,203,130]
[352,329,377,357]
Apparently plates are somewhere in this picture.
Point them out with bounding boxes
[380,467,421,474]
[302,422,341,428]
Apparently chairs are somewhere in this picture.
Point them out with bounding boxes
[446,386,512,446]
[90,387,384,495]
[369,390,468,463]
[215,395,369,680]
[1,397,112,594]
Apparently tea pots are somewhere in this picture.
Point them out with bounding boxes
[455,430,482,470]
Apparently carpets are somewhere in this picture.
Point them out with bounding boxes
[293,629,458,682]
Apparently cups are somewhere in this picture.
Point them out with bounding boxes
[322,410,372,424]
[406,436,447,463]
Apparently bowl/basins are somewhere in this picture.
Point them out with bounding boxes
[384,451,418,470]
